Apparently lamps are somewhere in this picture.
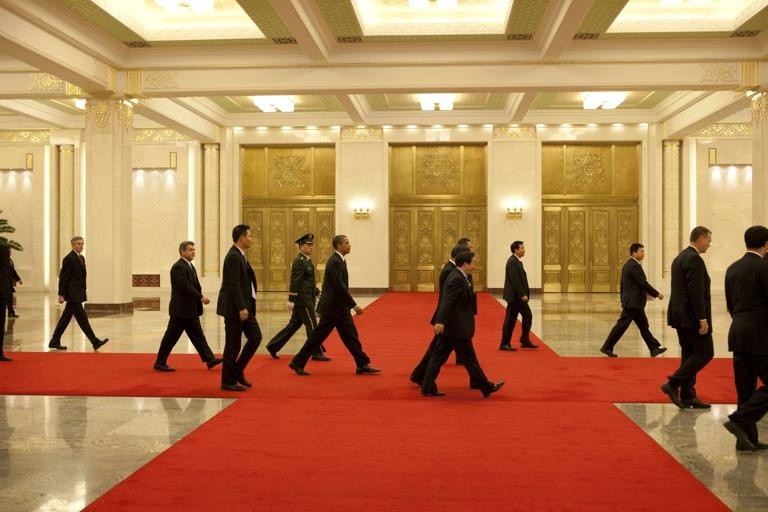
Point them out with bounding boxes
[350,197,372,220]
[503,199,527,220]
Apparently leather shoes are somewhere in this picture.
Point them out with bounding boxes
[600,348,616,357]
[153,345,380,392]
[521,341,538,348]
[409,357,505,398]
[500,344,516,350]
[660,382,767,450]
[49,344,66,349]
[649,348,666,356]
[93,338,108,349]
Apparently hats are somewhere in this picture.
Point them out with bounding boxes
[295,233,313,244]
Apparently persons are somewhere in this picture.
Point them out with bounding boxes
[661,225,715,409]
[622,401,767,511]
[723,224,767,451]
[266,233,331,362]
[600,243,668,357]
[409,238,505,398]
[57,394,89,450]
[0,243,11,361]
[159,395,208,445]
[0,394,15,490]
[288,235,382,376]
[499,240,539,351]
[6,246,23,316]
[3,315,24,353]
[153,240,224,372]
[48,236,110,351]
[217,223,263,392]
